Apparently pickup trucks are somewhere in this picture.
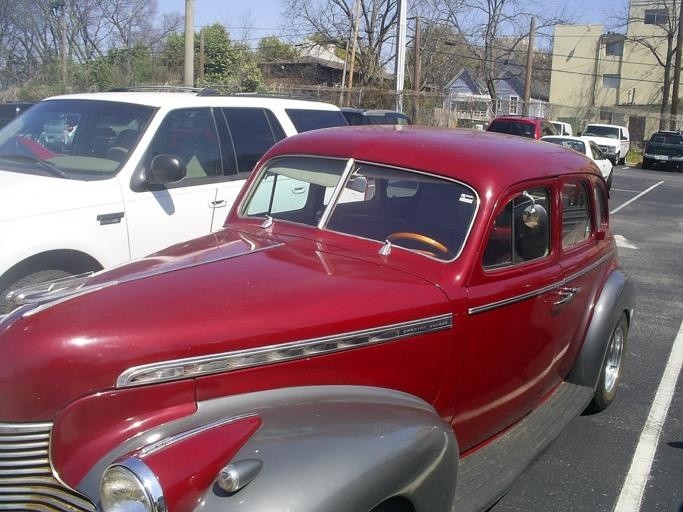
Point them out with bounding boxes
[642,129,683,173]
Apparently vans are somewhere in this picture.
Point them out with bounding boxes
[549,119,574,136]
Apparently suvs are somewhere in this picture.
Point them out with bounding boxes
[578,122,630,165]
[0,82,352,297]
[483,114,561,141]
[0,100,36,130]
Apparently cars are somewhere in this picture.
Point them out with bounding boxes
[0,123,635,511]
[340,105,412,127]
[537,135,613,193]
